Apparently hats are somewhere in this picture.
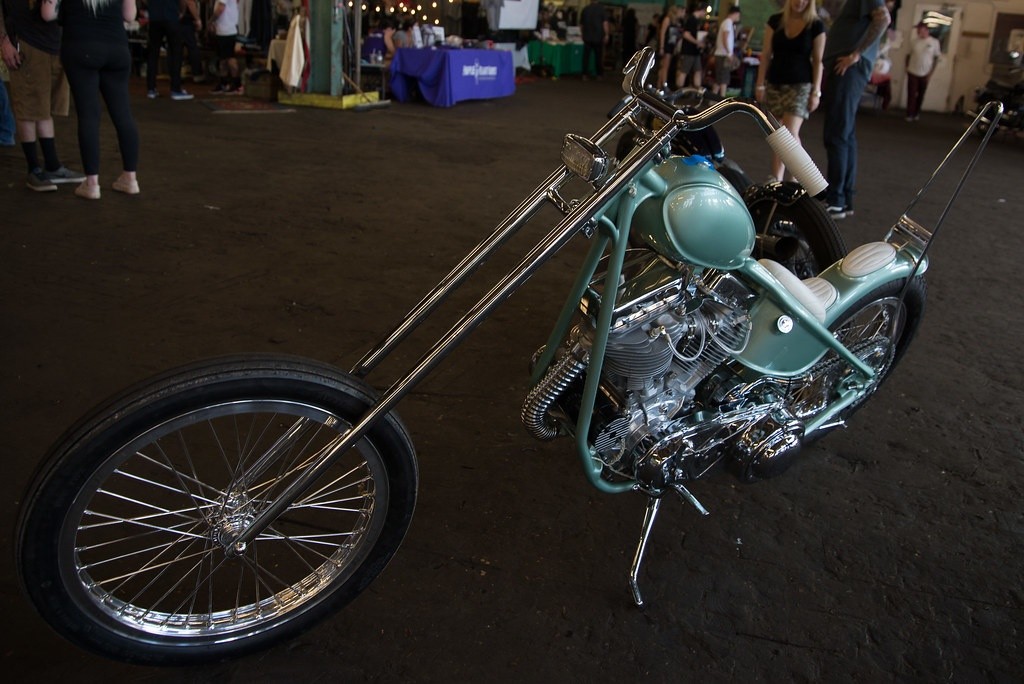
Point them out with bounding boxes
[913,20,927,28]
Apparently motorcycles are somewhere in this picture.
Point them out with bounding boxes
[9,44,1007,665]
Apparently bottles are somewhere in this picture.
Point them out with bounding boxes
[376,50,383,64]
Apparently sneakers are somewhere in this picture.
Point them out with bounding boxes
[26,169,58,191]
[43,166,87,183]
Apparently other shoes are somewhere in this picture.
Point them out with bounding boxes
[210,85,225,94]
[224,84,243,95]
[582,76,592,81]
[112,176,140,193]
[914,114,920,120]
[194,74,206,82]
[146,90,159,98]
[75,184,101,199]
[594,80,604,86]
[905,117,913,122]
[171,92,194,100]
[844,206,854,215]
[825,205,845,219]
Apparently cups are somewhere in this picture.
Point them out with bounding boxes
[369,54,377,63]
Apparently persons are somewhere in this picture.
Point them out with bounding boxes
[756,0,831,187]
[534,0,754,107]
[367,12,421,100]
[0,0,242,199]
[825,0,893,220]
[902,20,940,121]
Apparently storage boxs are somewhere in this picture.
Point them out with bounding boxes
[244,75,273,102]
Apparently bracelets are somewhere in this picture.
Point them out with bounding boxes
[757,85,765,90]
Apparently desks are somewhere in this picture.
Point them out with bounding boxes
[389,47,516,108]
[351,62,388,100]
[527,39,596,81]
[265,38,287,103]
